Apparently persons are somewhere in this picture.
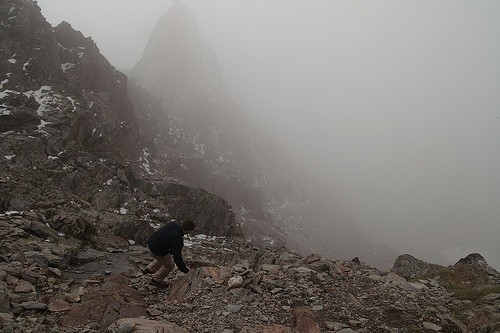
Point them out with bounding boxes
[143,219,196,286]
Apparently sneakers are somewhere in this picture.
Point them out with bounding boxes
[151,277,162,287]
[143,269,151,275]
